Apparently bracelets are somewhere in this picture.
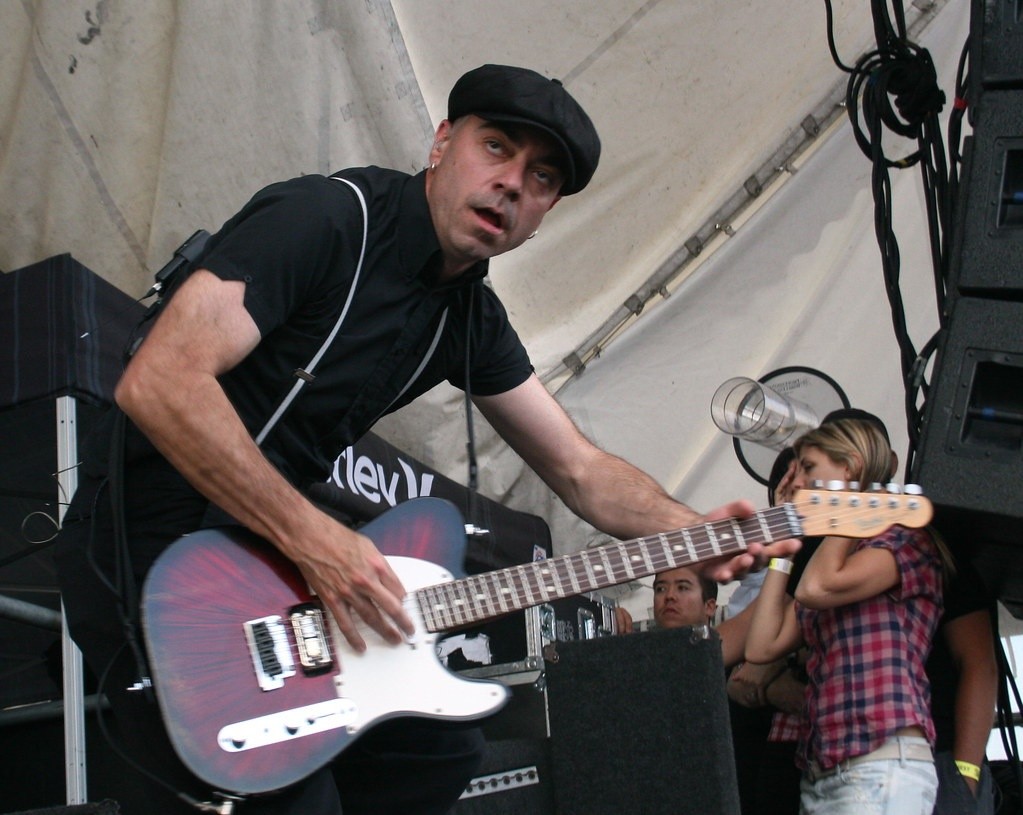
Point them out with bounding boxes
[955,760,981,782]
[768,558,794,575]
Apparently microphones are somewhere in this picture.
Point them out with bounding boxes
[144,229,212,299]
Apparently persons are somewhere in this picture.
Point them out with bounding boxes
[605,406,994,815]
[53,65,802,815]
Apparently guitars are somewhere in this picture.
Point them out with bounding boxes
[138,477,935,799]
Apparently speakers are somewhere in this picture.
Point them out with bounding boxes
[913,0,1023,520]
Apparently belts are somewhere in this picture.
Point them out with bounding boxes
[805,741,935,783]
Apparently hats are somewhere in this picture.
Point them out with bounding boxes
[448,63,601,197]
